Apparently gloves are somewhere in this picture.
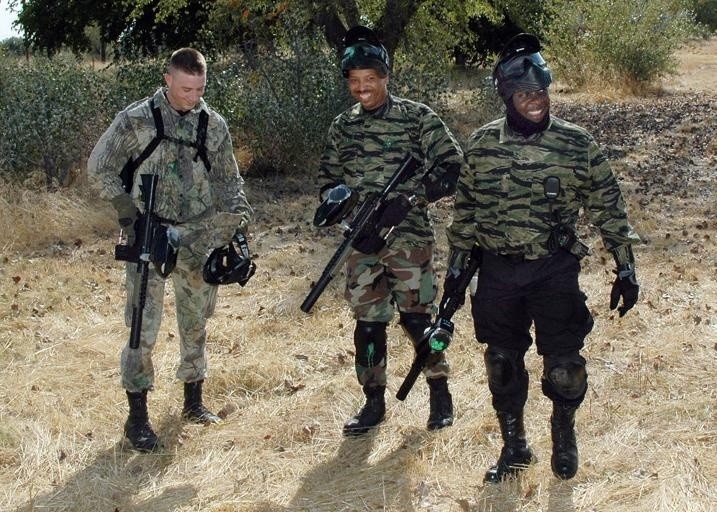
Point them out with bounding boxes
[112,193,140,246]
[377,194,412,231]
[440,274,466,304]
[609,277,641,318]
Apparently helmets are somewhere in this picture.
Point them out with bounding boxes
[339,25,389,73]
[489,33,551,100]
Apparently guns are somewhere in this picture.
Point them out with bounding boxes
[396,243,480,401]
[300,153,420,313]
[115,174,159,348]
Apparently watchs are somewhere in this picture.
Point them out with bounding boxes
[404,188,419,209]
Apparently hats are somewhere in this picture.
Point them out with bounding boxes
[207,210,243,249]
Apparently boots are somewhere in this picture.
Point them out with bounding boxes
[483,414,532,483]
[343,386,386,439]
[182,382,223,428]
[549,406,578,480]
[425,376,453,431]
[122,391,165,455]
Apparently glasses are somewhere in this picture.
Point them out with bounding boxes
[493,52,547,85]
[338,43,385,64]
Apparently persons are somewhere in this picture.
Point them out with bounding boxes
[443,35,637,483]
[84,44,249,453]
[313,33,465,435]
